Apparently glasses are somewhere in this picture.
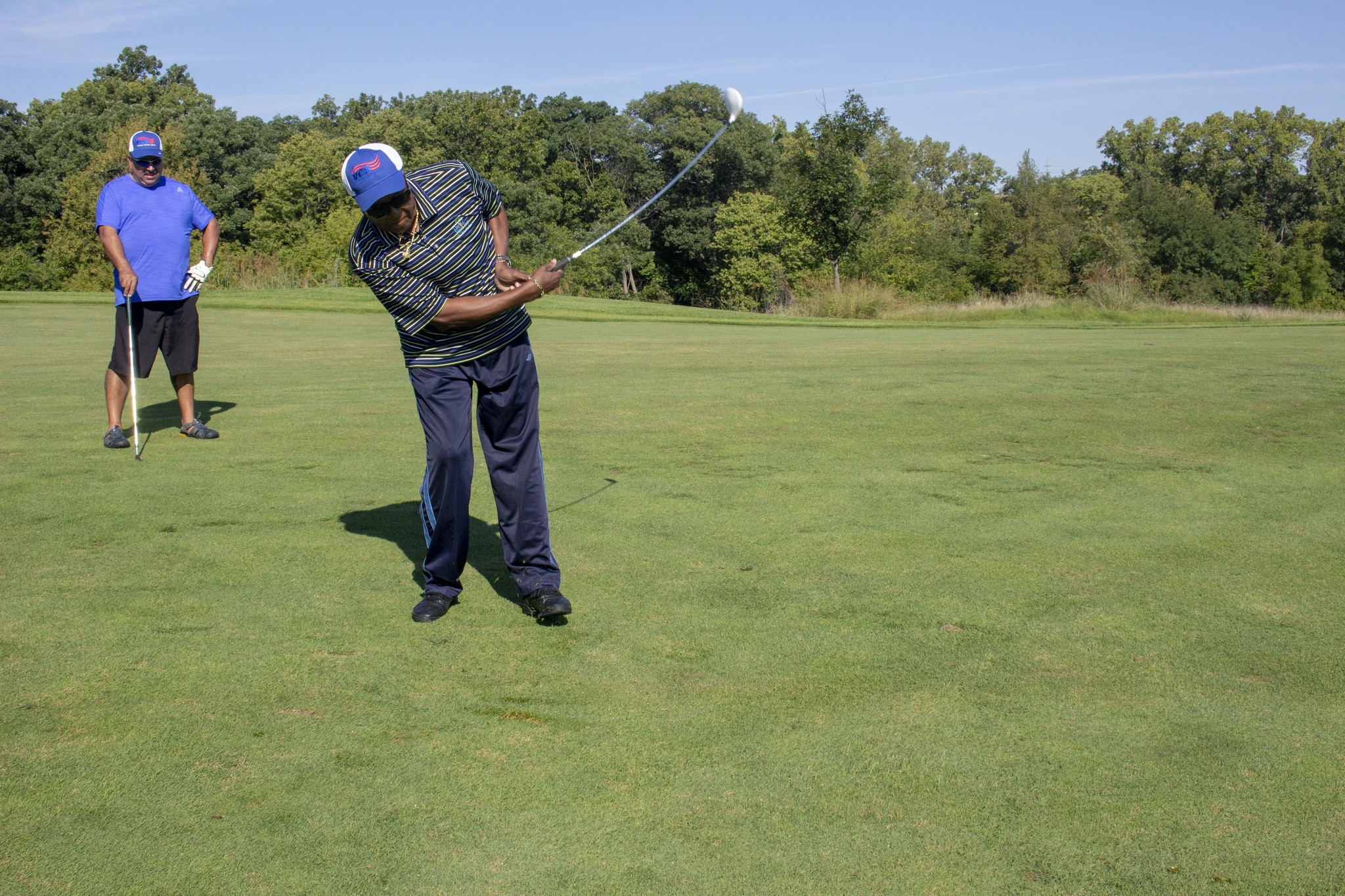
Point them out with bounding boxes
[130,156,163,167]
[366,175,411,219]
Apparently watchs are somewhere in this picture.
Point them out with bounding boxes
[496,254,511,265]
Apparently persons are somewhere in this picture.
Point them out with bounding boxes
[339,144,572,624]
[94,129,219,449]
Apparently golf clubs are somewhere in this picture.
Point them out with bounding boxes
[547,88,743,274]
[125,292,154,460]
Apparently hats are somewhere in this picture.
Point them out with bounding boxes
[128,130,163,158]
[341,143,403,213]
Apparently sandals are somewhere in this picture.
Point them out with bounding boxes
[181,411,219,439]
[104,422,129,448]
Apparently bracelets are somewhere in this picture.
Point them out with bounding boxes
[527,277,545,299]
[496,260,509,267]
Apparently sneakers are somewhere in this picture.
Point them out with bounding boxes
[523,586,573,617]
[411,593,454,622]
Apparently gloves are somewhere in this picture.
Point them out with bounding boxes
[183,260,214,294]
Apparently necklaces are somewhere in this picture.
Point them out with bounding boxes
[395,209,419,259]
